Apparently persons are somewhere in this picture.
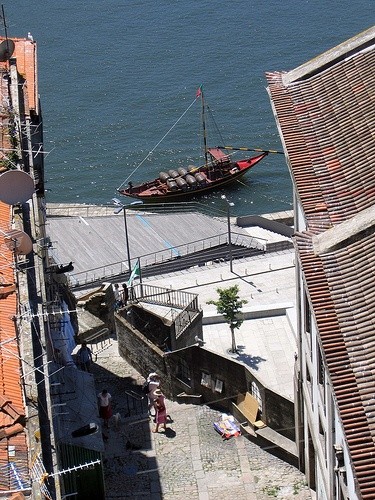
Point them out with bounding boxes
[77,342,93,373]
[122,283,129,306]
[142,372,161,415]
[152,389,166,433]
[96,389,112,429]
[114,283,121,311]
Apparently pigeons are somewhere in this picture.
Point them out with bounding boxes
[195,336,207,344]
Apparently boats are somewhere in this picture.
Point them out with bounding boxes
[117,84,269,204]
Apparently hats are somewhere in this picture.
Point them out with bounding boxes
[149,372,157,379]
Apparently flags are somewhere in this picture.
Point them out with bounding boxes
[196,88,201,96]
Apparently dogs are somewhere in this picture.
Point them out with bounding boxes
[111,413,121,425]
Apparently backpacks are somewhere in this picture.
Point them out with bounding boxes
[142,381,155,394]
